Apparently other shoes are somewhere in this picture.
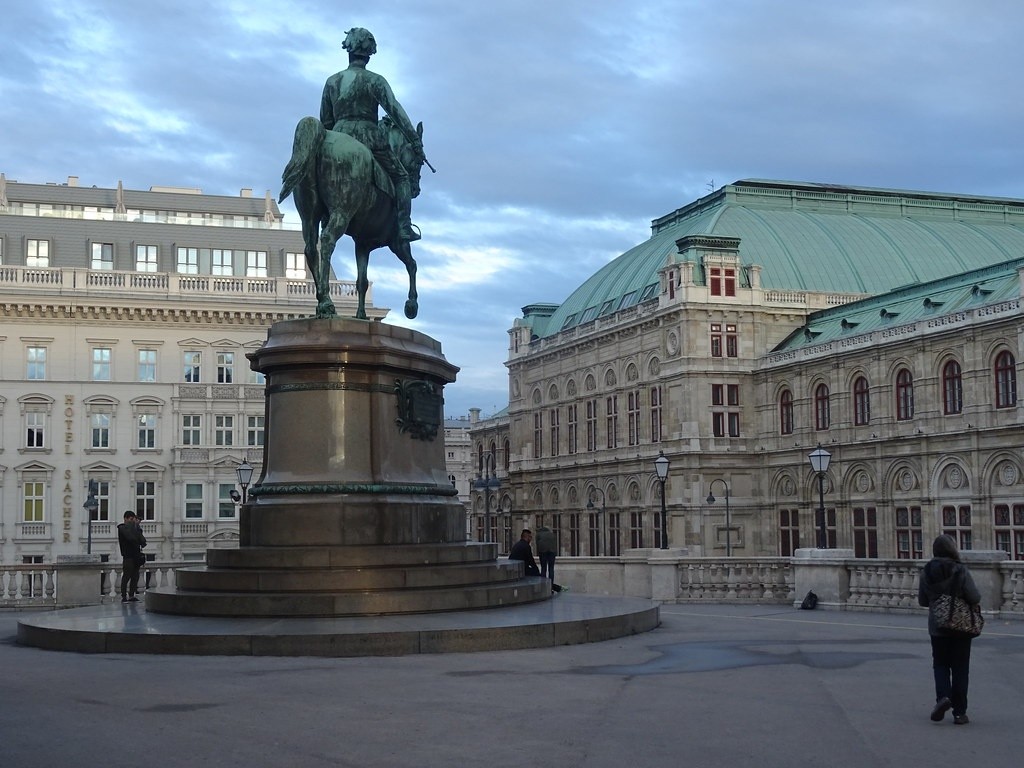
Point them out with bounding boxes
[560,587,569,592]
[129,597,139,601]
[122,598,127,601]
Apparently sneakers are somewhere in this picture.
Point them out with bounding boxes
[954,714,969,724]
[931,697,950,721]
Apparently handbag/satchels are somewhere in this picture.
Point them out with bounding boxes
[135,553,145,567]
[931,594,985,639]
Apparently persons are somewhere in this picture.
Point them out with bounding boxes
[509,529,567,592]
[117,511,147,604]
[921,535,981,722]
[320,23,427,239]
[535,524,556,590]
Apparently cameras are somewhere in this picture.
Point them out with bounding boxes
[135,515,143,521]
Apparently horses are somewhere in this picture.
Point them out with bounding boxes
[278,116,425,319]
